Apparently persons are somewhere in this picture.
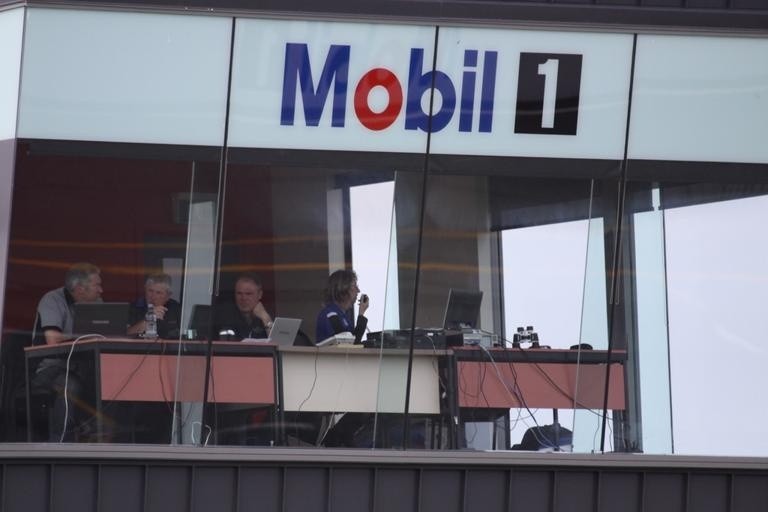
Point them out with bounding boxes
[315,268,369,346]
[213,272,275,340]
[126,274,179,339]
[25,261,106,442]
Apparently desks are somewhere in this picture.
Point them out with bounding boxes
[450,344,629,449]
[21,341,280,444]
[279,346,450,447]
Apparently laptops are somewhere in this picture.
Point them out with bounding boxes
[55,302,130,342]
[241,317,305,347]
[161,305,218,341]
[419,289,483,332]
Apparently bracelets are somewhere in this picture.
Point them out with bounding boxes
[264,319,273,329]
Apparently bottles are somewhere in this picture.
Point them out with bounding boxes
[145,304,157,339]
